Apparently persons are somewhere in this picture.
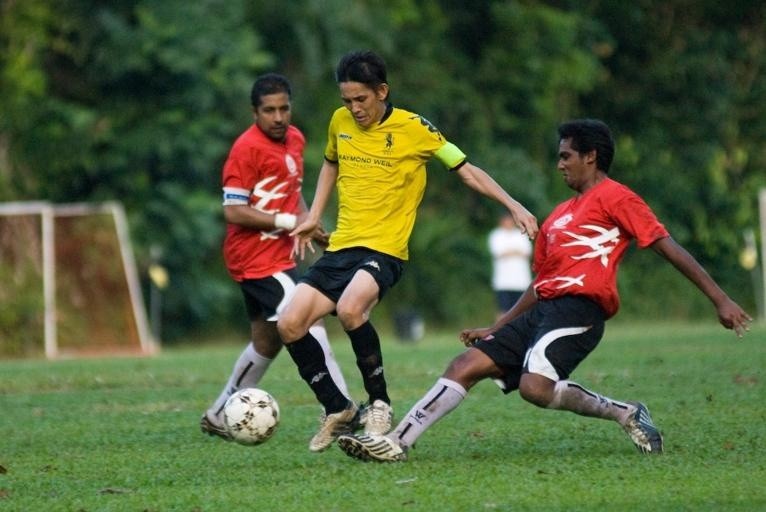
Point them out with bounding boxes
[199,74,356,440]
[337,120,752,458]
[273,50,539,452]
[487,213,535,317]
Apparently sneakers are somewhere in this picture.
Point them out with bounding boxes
[308,401,360,452]
[362,399,393,436]
[621,401,663,456]
[199,409,233,442]
[338,434,408,463]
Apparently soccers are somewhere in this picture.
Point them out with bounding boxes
[223,390,279,446]
